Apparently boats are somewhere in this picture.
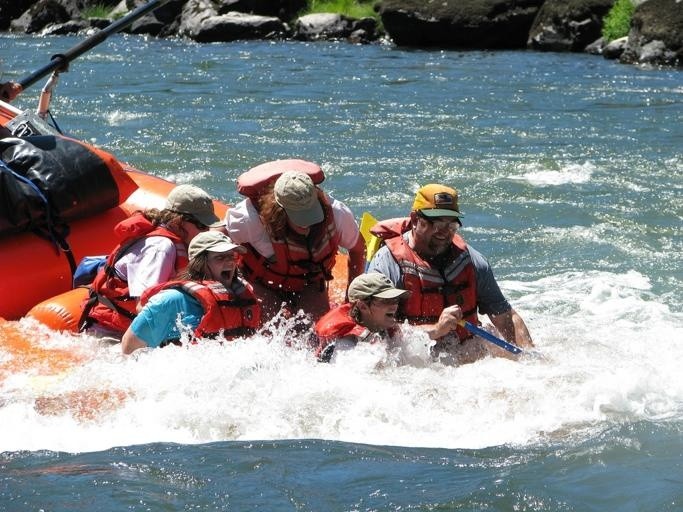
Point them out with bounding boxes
[0,100,363,443]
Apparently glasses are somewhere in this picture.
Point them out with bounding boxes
[417,212,461,230]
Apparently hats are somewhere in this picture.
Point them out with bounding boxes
[274,170,324,226]
[347,273,409,303]
[187,231,247,260]
[164,184,226,228]
[412,184,463,218]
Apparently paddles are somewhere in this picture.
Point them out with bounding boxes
[359,212,384,275]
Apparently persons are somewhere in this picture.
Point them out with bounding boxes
[367,182,534,348]
[313,271,409,362]
[78,182,218,341]
[207,171,366,333]
[120,231,259,356]
